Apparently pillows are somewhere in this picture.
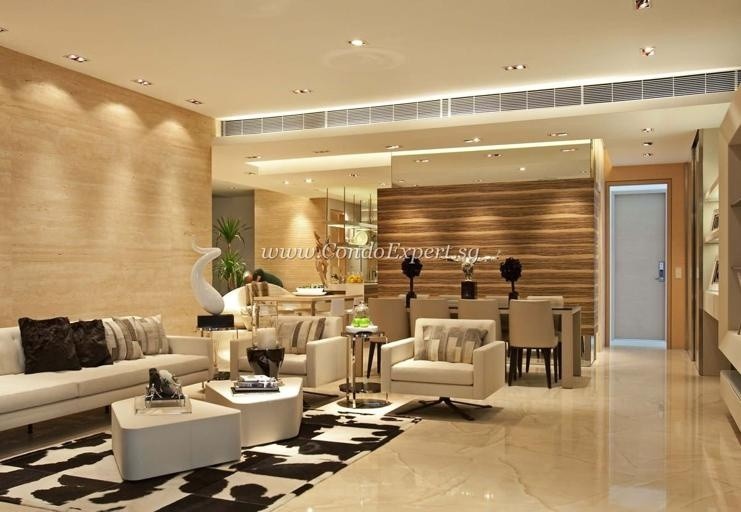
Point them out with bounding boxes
[18,313,170,375]
[277,318,327,355]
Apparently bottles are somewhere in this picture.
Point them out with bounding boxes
[352,300,370,328]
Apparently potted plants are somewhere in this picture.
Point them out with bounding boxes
[500,257,522,301]
[401,254,423,308]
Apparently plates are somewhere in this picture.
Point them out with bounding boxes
[291,291,327,296]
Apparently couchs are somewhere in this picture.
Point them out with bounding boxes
[0,316,212,434]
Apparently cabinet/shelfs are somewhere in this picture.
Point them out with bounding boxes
[324,187,377,250]
[703,83,741,433]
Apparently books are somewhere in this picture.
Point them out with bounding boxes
[232,374,279,393]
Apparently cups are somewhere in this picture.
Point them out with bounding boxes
[247,347,284,384]
[251,304,279,349]
[337,270,364,284]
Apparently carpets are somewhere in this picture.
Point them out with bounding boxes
[0,412,422,512]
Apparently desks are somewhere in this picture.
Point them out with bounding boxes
[254,294,362,328]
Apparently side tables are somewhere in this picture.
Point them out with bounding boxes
[338,331,391,409]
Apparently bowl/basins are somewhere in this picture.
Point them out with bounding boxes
[296,284,324,293]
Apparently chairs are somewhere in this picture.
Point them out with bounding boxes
[354,293,582,421]
[229,316,352,388]
